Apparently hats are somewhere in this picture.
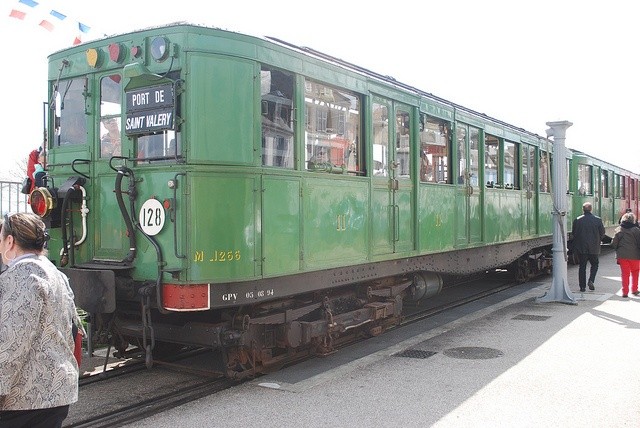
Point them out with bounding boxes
[626,208,630,211]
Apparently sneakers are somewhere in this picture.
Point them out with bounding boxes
[623,295,628,297]
[632,290,640,296]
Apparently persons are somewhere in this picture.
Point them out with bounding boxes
[99,115,121,156]
[571,202,605,291]
[619,208,639,227]
[610,212,640,298]
[0,212,86,427]
[307,142,336,171]
[167,139,175,156]
[26,129,49,204]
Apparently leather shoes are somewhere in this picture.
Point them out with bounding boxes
[580,288,585,292]
[588,281,594,290]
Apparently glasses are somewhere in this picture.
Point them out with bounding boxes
[314,151,328,156]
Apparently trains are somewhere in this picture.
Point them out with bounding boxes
[30,25,640,383]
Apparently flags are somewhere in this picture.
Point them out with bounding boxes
[16,0,36,29]
[40,7,67,36]
[70,18,100,49]
